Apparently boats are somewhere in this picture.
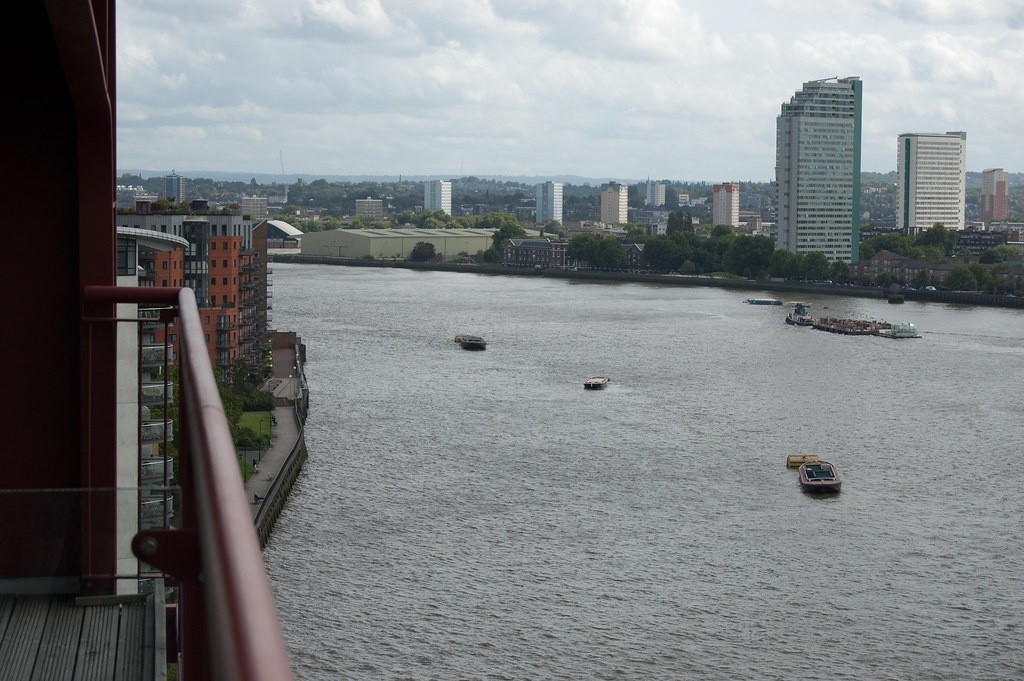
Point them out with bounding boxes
[817,315,923,338]
[746,297,782,305]
[461,333,486,349]
[584,373,608,389]
[785,302,815,326]
[798,460,842,492]
